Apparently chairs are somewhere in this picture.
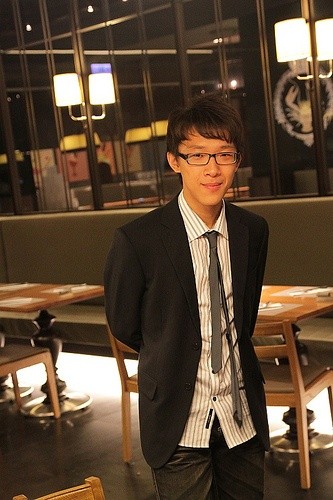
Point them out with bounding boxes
[252,320,333,490]
[12,476,105,500]
[105,317,140,465]
[0,344,62,420]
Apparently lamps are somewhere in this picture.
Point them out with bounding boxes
[89,72,116,120]
[59,132,101,158]
[124,119,168,146]
[53,72,88,129]
[274,17,315,91]
[315,19,333,80]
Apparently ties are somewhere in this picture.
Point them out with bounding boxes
[203,231,242,429]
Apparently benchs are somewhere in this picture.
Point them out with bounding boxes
[0,197,333,365]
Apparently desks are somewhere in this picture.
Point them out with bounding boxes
[0,282,104,418]
[258,285,333,461]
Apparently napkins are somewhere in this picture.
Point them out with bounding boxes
[258,302,283,312]
[290,287,329,296]
[0,298,32,306]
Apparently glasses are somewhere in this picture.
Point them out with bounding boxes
[175,151,241,166]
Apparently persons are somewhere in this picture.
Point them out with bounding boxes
[102,95,271,500]
